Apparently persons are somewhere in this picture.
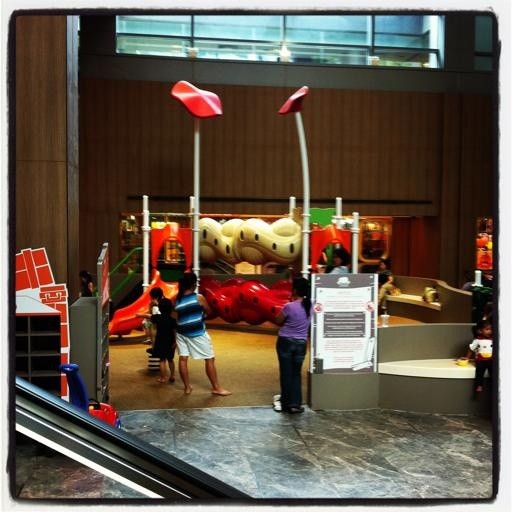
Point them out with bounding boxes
[274,278,313,413]
[142,287,164,344]
[462,320,494,393]
[136,298,177,384]
[79,269,94,297]
[375,259,394,311]
[174,273,232,396]
[329,249,349,273]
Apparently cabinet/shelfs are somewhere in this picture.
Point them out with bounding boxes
[16,246,70,405]
[70,242,111,405]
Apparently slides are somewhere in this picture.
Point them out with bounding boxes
[108,269,191,337]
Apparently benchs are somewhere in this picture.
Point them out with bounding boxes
[378,324,494,417]
[384,274,474,323]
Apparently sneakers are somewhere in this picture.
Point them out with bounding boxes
[271,394,305,414]
